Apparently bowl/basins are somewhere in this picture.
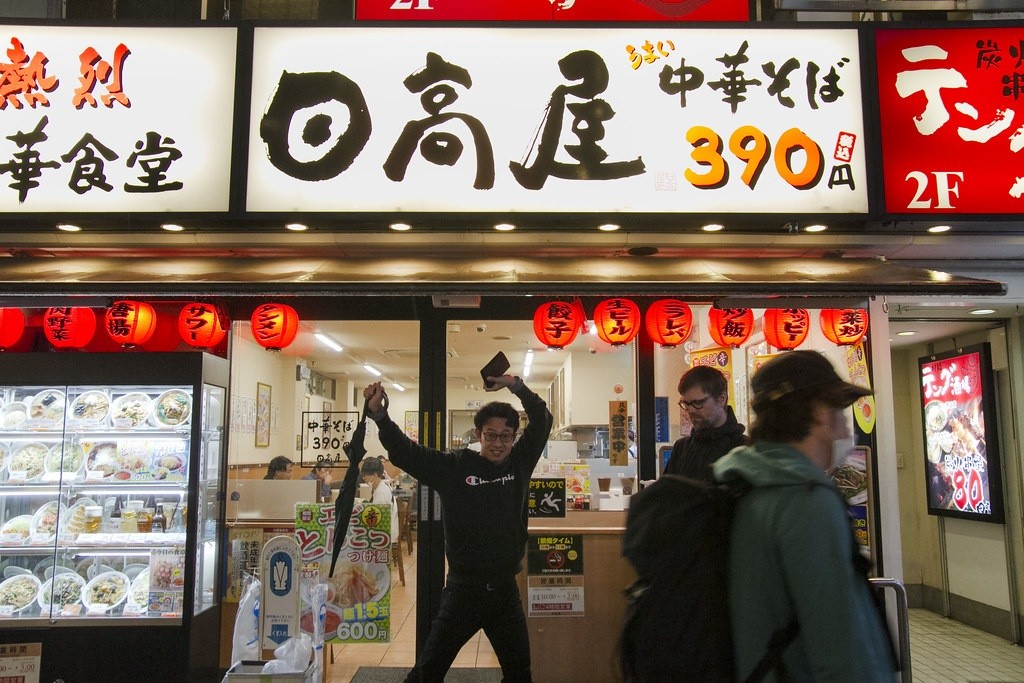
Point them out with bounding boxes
[565,469,585,493]
[861,404,872,422]
[299,548,391,641]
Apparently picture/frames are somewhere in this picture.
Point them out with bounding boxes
[255,382,272,447]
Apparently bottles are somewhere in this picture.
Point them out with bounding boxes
[566,497,589,510]
[110,496,123,530]
[152,503,166,533]
[583,476,590,493]
[145,495,156,508]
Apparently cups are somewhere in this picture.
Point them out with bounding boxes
[597,477,611,491]
[84,506,103,533]
[135,507,155,532]
[330,489,339,503]
[359,484,372,500]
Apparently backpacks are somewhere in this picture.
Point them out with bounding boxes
[617,474,862,683]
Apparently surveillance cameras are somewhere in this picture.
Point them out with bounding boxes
[475,324,486,333]
[589,347,597,354]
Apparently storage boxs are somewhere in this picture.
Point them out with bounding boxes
[226,659,316,683]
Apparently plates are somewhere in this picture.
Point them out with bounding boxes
[853,378,868,408]
[842,456,868,507]
[924,400,954,464]
[172,577,184,588]
[149,604,161,611]
[0,388,193,614]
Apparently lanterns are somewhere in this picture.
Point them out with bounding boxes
[708,307,753,349]
[179,302,226,346]
[43,307,96,351]
[251,303,299,353]
[0,308,24,351]
[645,299,693,348]
[762,307,810,351]
[105,301,156,349]
[534,301,581,350]
[819,308,868,347]
[593,298,641,346]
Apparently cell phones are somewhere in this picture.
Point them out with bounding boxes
[480,351,510,388]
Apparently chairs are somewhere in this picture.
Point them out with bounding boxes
[390,493,414,587]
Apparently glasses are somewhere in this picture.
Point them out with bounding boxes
[480,431,515,443]
[678,390,720,410]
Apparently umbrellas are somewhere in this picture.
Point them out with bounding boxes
[328,390,389,577]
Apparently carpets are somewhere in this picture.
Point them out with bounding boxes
[348,666,505,683]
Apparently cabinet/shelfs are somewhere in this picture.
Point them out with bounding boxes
[0,351,230,683]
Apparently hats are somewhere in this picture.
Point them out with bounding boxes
[753,350,872,409]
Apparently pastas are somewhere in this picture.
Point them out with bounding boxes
[9,394,189,481]
[0,576,124,614]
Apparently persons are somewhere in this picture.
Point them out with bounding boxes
[616,350,900,683]
[302,460,335,496]
[377,455,387,475]
[363,375,555,683]
[361,456,400,542]
[263,456,294,479]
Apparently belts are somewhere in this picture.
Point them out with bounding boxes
[449,575,516,591]
